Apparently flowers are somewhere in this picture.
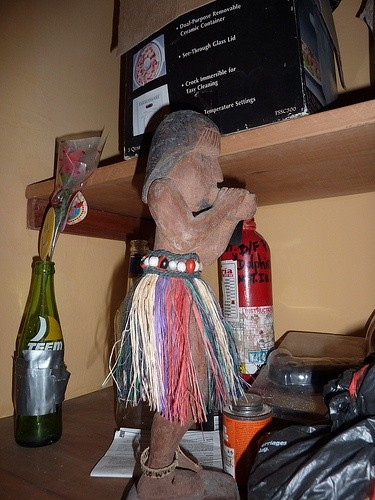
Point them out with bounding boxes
[33,147,87,332]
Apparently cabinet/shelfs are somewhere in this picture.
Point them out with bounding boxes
[0,97,375,500]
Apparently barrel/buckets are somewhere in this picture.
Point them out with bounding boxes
[222,404,274,481]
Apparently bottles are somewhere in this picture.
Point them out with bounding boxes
[218,217,275,382]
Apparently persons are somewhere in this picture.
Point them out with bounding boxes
[107,110,256,500]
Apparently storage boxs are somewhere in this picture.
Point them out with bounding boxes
[112,1,342,161]
